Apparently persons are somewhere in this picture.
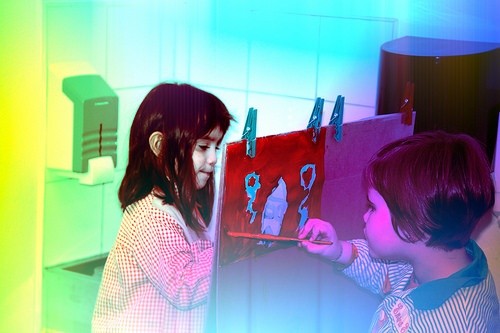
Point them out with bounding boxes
[297,132,500,333]
[91,83,239,333]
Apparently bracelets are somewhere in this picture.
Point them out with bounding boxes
[331,242,344,262]
